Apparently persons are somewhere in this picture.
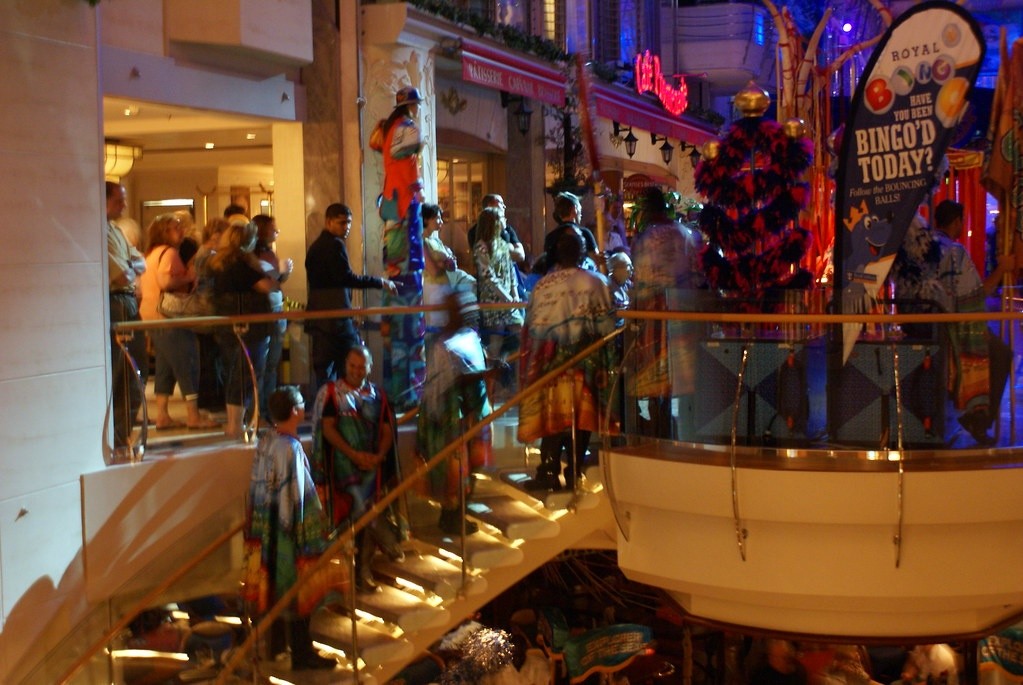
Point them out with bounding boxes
[419,189,710,532]
[510,549,1023,685]
[247,386,339,669]
[105,181,293,444]
[515,226,614,497]
[935,199,1016,444]
[369,84,424,285]
[310,345,405,590]
[303,204,404,385]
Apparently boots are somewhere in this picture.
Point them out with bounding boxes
[267,613,290,661]
[288,615,338,672]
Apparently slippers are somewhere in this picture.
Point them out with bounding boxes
[188,420,224,429]
[156,419,185,431]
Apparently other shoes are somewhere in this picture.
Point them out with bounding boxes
[536,474,562,493]
[439,499,484,535]
[134,419,151,427]
[563,464,582,489]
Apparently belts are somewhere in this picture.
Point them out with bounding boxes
[110,288,137,297]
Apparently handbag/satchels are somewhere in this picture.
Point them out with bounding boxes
[157,244,189,317]
[184,254,216,333]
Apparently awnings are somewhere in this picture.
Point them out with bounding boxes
[439,32,568,107]
[589,77,721,146]
